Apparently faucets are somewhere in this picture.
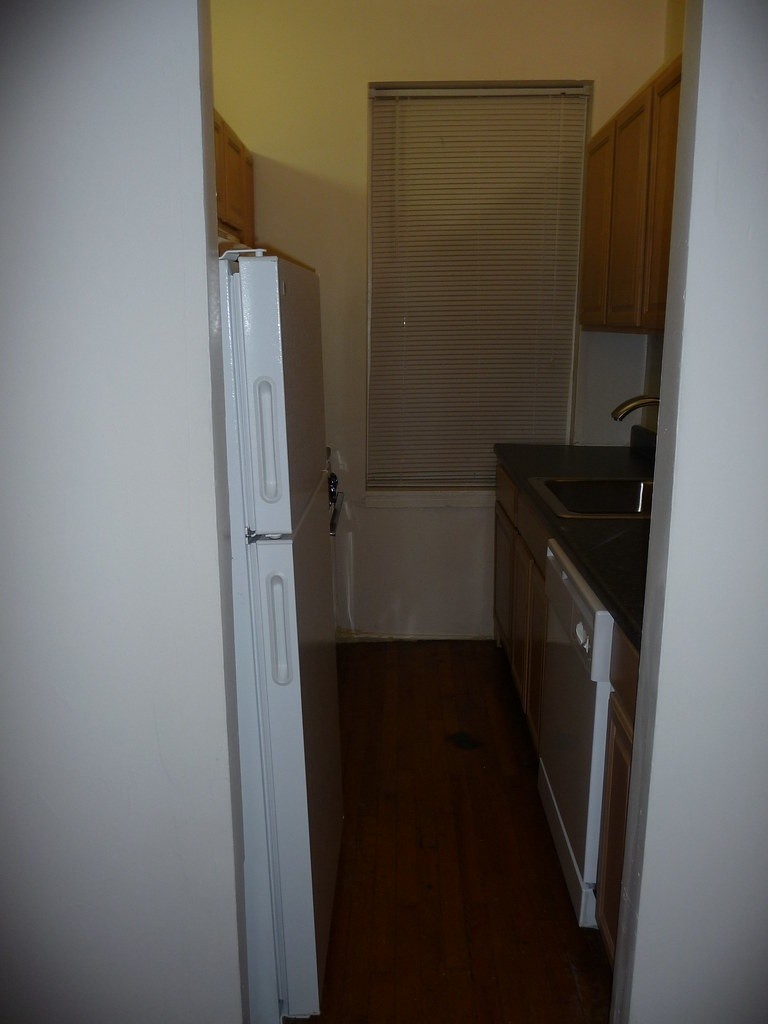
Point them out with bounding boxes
[611,396,660,422]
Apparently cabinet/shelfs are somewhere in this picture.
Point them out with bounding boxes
[507,493,561,757]
[640,54,683,334]
[213,109,255,250]
[577,83,654,334]
[491,462,520,647]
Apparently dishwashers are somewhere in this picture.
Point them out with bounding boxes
[536,538,614,929]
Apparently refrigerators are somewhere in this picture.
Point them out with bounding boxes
[215,247,345,1024]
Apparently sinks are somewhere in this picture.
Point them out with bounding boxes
[527,476,653,519]
